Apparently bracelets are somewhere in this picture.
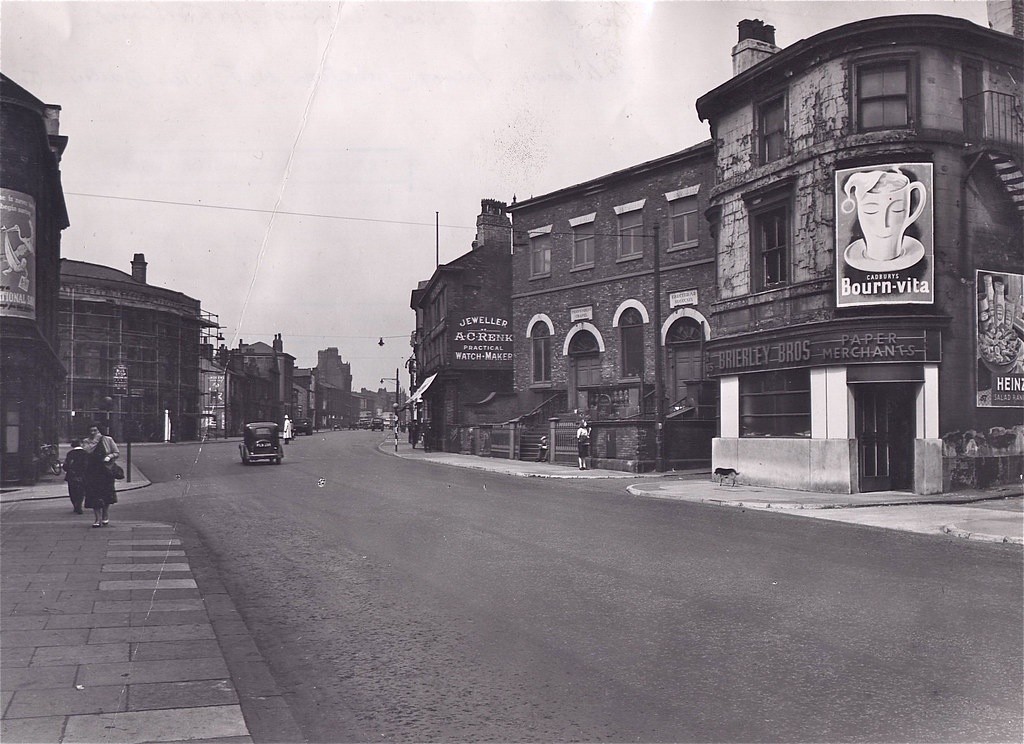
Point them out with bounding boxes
[107,455,112,462]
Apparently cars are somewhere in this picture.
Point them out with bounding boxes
[347,417,384,431]
[239,422,284,465]
[291,417,312,436]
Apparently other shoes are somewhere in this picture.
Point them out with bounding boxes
[535,459,540,462]
[74,507,83,514]
[542,459,546,462]
[580,467,583,470]
[93,523,100,527]
[102,519,108,524]
[583,467,589,470]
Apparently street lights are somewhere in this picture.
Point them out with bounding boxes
[380,367,399,404]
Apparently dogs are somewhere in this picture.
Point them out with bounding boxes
[714,468,740,487]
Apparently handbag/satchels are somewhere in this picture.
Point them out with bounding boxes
[112,463,125,480]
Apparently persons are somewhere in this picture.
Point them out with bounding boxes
[282,414,294,445]
[534,436,549,462]
[62,437,85,514]
[577,419,592,470]
[82,422,120,527]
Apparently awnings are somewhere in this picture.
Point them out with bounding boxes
[405,371,441,404]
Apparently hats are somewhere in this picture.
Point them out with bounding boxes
[284,414,288,418]
[540,436,546,440]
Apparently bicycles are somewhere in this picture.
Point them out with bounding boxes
[40,443,62,475]
